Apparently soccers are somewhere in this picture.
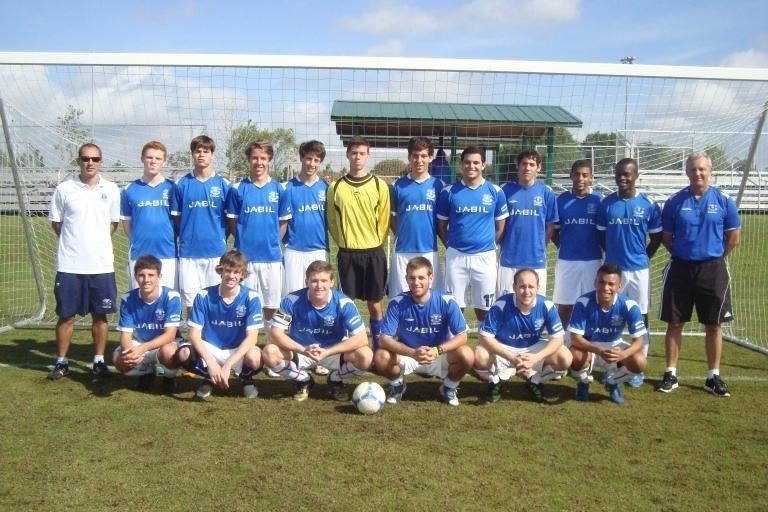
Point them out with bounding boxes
[353,382,387,415]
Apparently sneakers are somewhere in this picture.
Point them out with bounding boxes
[628,372,645,388]
[137,366,176,394]
[525,376,544,402]
[488,380,502,402]
[196,378,213,398]
[575,380,592,402]
[604,382,624,404]
[47,363,69,379]
[268,367,350,401]
[240,375,258,398]
[92,362,113,378]
[657,371,678,393]
[387,382,407,404]
[704,374,731,397]
[439,383,459,406]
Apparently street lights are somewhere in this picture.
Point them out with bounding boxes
[618,55,637,159]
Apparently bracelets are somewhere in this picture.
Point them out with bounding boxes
[436,344,443,355]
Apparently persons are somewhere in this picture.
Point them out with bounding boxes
[473,268,572,407]
[595,158,663,389]
[551,159,606,327]
[561,262,648,405]
[372,256,474,408]
[435,145,509,326]
[47,142,123,380]
[282,140,331,294]
[656,150,742,397]
[429,149,451,185]
[222,139,293,325]
[497,147,561,302]
[111,253,182,393]
[326,137,391,351]
[121,140,178,290]
[259,260,377,403]
[385,136,448,294]
[175,250,263,399]
[169,134,230,320]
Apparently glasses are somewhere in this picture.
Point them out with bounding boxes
[81,156,101,162]
